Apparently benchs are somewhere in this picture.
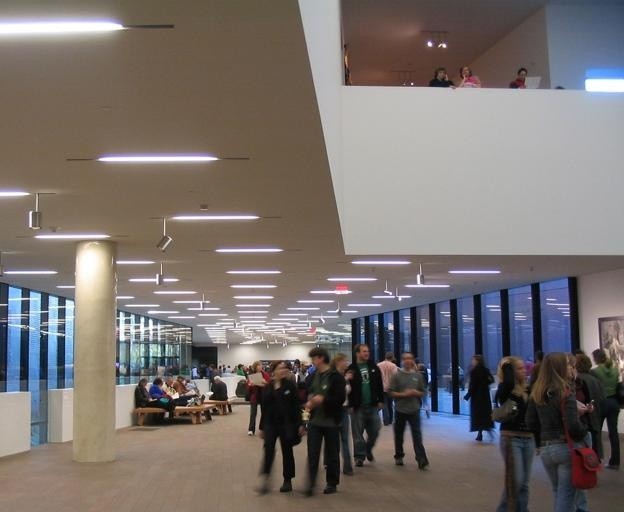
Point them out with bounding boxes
[133,399,234,426]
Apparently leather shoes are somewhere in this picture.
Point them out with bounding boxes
[604,463,619,469]
[476,434,482,440]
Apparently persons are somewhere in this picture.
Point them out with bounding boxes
[255,360,305,493]
[429,68,455,87]
[162,377,194,406]
[388,351,429,468]
[376,351,400,425]
[208,364,221,391]
[457,65,481,88]
[568,353,594,512]
[528,351,546,447]
[185,379,205,403]
[575,354,605,464]
[526,352,588,512]
[494,356,540,512]
[173,376,199,405]
[511,69,528,88]
[415,358,429,418]
[219,364,254,376]
[458,366,465,391]
[465,354,495,440]
[325,353,354,475]
[245,360,270,436]
[209,375,232,415]
[135,379,173,423]
[192,364,208,379]
[149,378,174,405]
[265,359,315,377]
[305,347,346,497]
[444,362,453,392]
[590,350,622,469]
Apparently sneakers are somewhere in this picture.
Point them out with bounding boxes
[356,458,363,467]
[324,483,336,493]
[418,456,428,468]
[397,458,403,465]
[366,450,374,461]
[247,431,254,436]
[280,485,292,492]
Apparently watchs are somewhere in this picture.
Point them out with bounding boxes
[344,343,384,467]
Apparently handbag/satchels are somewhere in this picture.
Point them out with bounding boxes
[491,395,519,422]
[570,447,601,490]
[615,381,624,409]
